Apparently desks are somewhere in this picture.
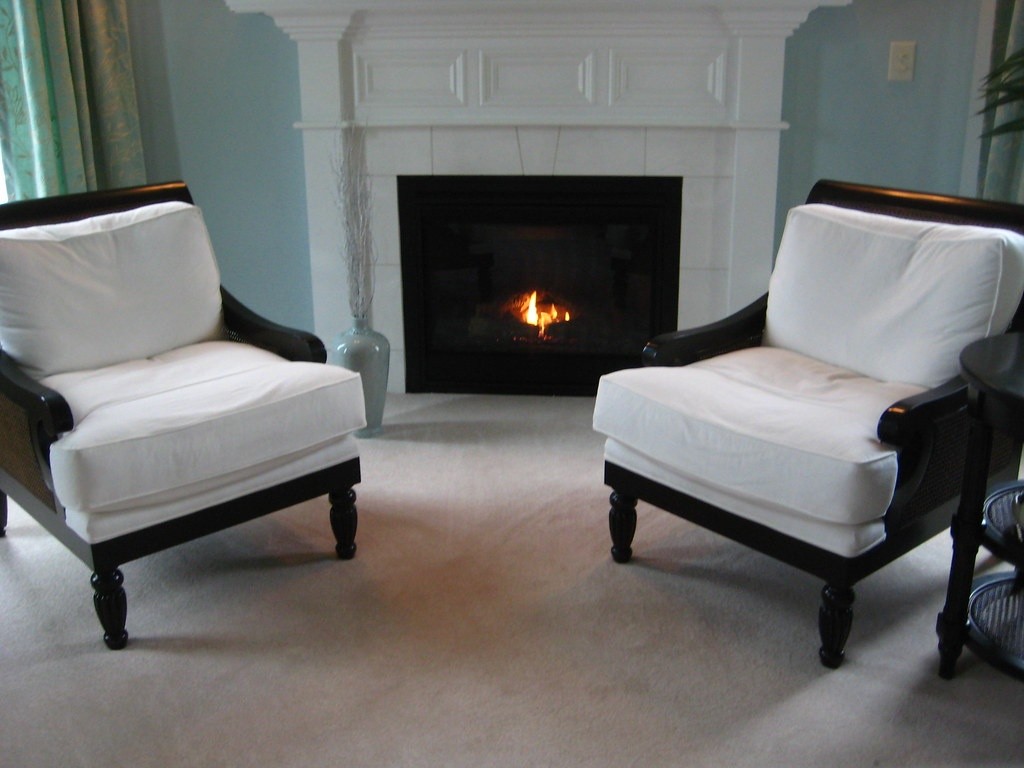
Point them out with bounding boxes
[934,332,1024,685]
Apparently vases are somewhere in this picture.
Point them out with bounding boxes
[331,315,392,437]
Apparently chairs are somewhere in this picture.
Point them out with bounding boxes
[0,181,367,651]
[593,178,1022,669]
